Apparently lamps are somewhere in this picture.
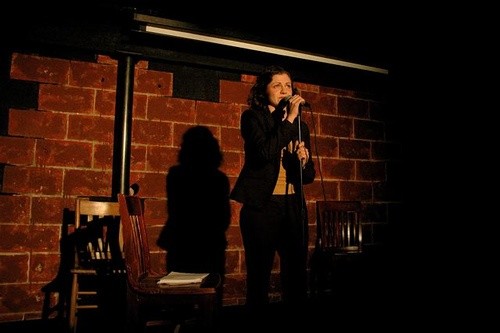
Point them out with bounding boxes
[136,24,390,76]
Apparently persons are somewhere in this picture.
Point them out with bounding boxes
[226,68,319,319]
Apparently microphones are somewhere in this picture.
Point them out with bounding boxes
[284,94,310,107]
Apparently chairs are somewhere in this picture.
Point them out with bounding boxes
[117,194,220,333]
[68,198,125,331]
[315,200,369,328]
